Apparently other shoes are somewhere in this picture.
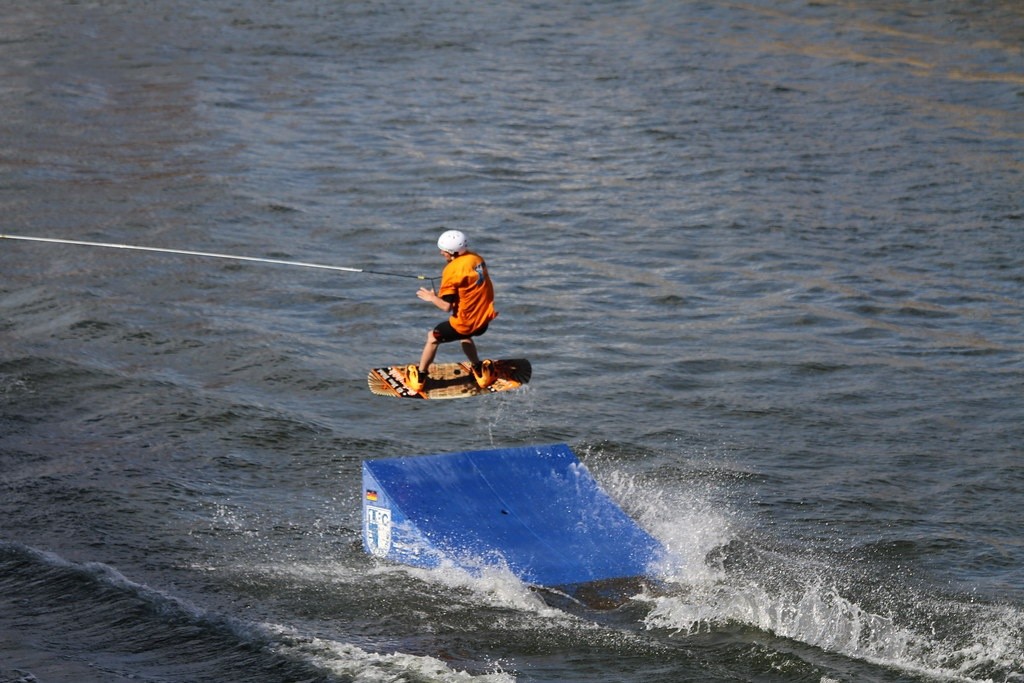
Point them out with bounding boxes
[472,362,494,378]
[406,366,428,383]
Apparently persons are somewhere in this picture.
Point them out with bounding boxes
[406,230,499,392]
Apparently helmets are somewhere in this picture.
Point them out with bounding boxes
[437,230,467,256]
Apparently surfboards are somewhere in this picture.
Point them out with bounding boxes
[367,358,532,400]
